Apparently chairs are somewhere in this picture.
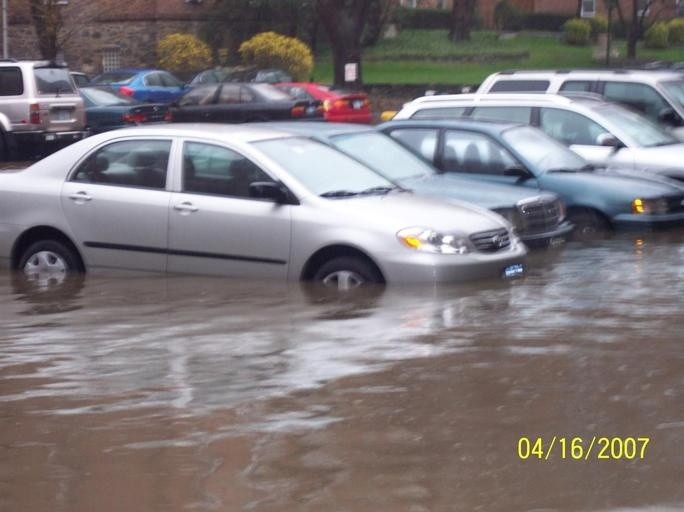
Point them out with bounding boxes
[441,145,463,173]
[93,158,115,183]
[228,158,256,197]
[464,143,488,172]
[183,153,210,192]
[127,150,160,187]
